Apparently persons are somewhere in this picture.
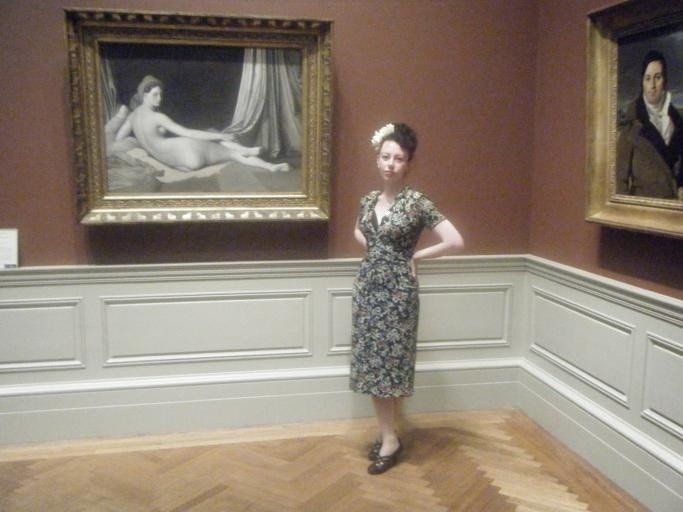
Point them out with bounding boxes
[348,121,465,475]
[617,49,683,203]
[115,75,294,174]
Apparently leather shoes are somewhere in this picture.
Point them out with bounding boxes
[368,436,402,475]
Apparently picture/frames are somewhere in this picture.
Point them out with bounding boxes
[584,0,683,242]
[61,7,334,226]
